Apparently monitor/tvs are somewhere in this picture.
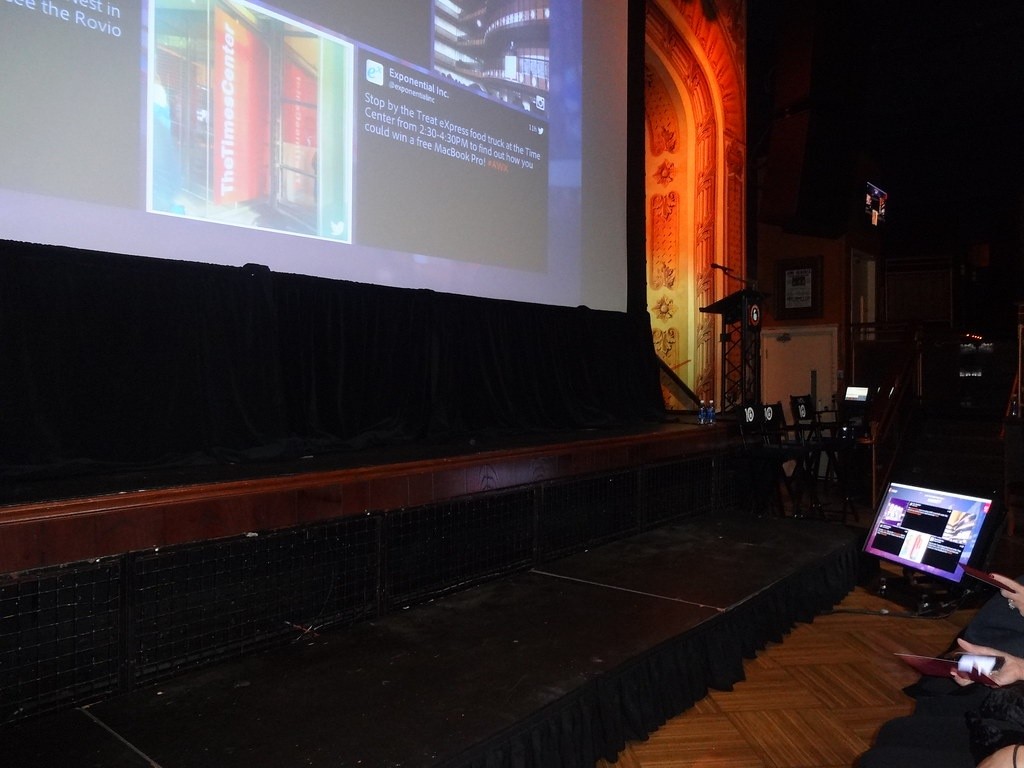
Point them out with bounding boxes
[844,387,868,401]
[863,482,1003,585]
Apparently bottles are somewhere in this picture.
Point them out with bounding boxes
[698,400,706,424]
[706,400,715,423]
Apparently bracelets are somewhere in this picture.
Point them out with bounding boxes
[1013,742,1022,768]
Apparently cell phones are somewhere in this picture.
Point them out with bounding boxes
[951,652,1006,680]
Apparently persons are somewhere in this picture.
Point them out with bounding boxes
[855,636,1024,768]
[951,571,1024,668]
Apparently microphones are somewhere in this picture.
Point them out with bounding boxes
[710,262,734,272]
